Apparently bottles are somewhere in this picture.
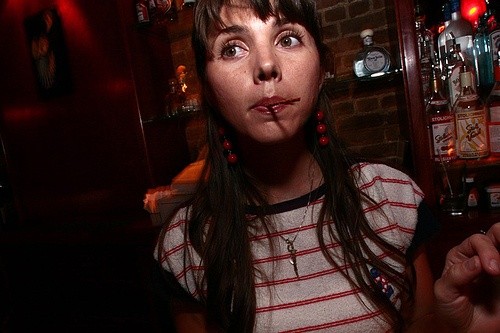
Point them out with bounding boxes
[413,0,500,163]
[134,0,196,24]
[351,29,399,94]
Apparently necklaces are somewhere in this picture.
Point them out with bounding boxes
[262,159,316,278]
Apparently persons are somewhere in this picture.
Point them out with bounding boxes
[151,0,500,333]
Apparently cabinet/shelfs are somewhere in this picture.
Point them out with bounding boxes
[393,0,500,231]
[135,0,400,129]
